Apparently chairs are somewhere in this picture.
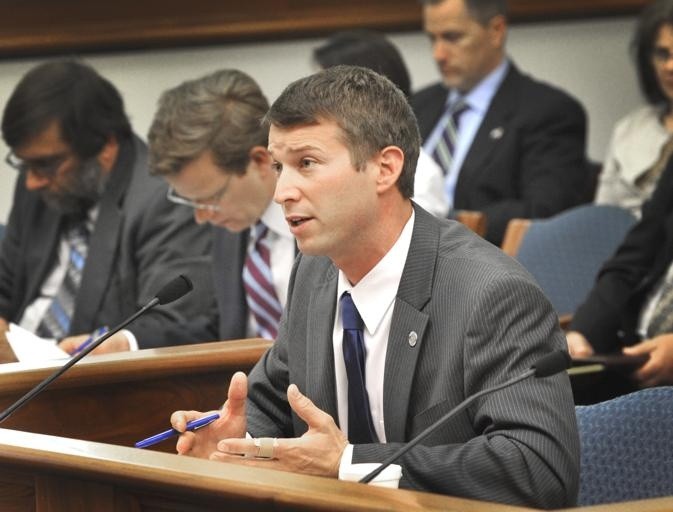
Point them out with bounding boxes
[575,384,672,508]
[446,210,487,238]
[500,204,638,328]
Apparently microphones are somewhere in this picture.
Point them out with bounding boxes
[358,348,572,486]
[0,273,194,427]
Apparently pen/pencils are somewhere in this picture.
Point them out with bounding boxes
[70,325,109,356]
[134,415,221,449]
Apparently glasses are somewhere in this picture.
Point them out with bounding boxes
[167,171,231,211]
[5,150,67,175]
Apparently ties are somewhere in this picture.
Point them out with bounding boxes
[241,221,282,340]
[341,293,380,444]
[431,100,471,176]
[33,220,89,337]
[646,261,673,338]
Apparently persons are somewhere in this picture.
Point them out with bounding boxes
[2,61,223,362]
[55,67,300,357]
[595,0,673,217]
[565,157,673,386]
[168,62,579,509]
[410,0,588,246]
[311,30,449,217]
